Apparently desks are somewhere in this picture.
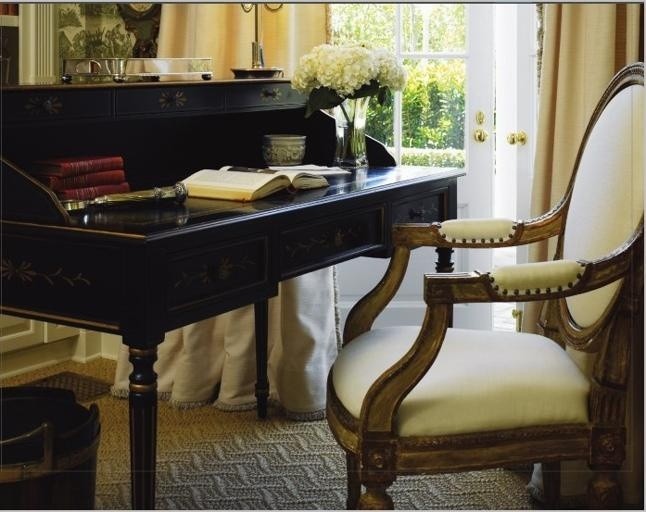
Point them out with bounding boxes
[0,83,466,510]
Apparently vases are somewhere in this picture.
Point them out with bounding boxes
[334,126,369,169]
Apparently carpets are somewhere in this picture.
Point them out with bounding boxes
[1,353,547,509]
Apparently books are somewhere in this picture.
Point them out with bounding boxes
[28,154,124,178]
[35,170,125,191]
[55,182,131,199]
[181,165,331,203]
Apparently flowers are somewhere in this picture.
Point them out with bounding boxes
[291,37,402,126]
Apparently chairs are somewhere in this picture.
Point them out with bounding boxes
[327,59,645,508]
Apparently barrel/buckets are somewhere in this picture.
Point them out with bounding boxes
[0,387,100,510]
